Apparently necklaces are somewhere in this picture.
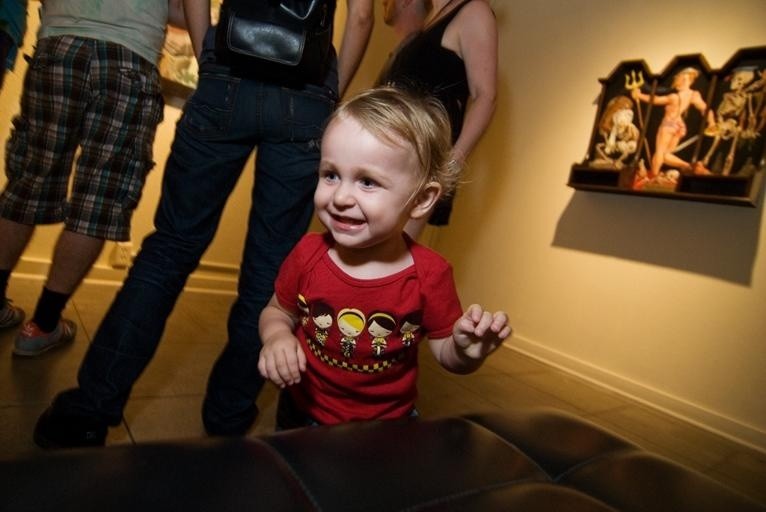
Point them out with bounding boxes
[421,1,451,30]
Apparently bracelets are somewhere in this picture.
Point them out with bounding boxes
[447,156,465,178]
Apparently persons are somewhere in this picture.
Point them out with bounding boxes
[1,0,193,358]
[1,0,30,99]
[598,95,642,168]
[28,0,378,445]
[252,83,511,433]
[375,0,500,249]
[636,66,713,176]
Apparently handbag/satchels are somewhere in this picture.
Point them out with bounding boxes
[215,0,338,92]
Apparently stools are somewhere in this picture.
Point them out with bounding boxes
[0,404,766,512]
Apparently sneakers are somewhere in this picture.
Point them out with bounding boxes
[205,401,260,439]
[13,319,78,356]
[1,300,27,329]
[33,386,109,450]
